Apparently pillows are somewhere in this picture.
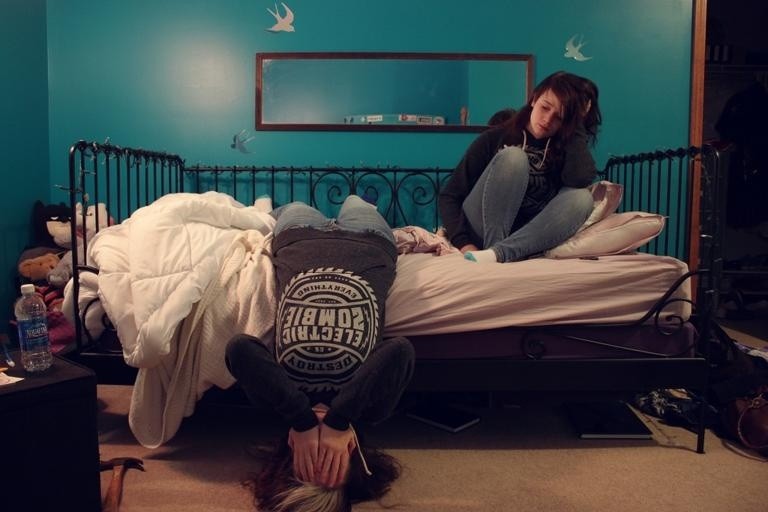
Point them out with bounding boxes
[546,179,670,258]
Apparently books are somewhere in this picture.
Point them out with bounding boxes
[406,412,480,433]
[572,401,654,441]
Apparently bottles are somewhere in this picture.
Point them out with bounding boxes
[15,284,53,371]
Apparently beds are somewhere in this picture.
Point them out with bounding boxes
[68,140,722,453]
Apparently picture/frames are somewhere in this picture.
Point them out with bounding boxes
[254,52,536,134]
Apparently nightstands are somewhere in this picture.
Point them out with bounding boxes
[1,349,104,511]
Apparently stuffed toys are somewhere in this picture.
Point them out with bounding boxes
[20,201,113,352]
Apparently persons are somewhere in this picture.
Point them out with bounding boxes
[436,70,603,262]
[224,195,417,512]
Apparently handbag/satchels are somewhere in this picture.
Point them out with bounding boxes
[726,391,767,456]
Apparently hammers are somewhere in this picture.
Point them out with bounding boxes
[96,456,145,512]
